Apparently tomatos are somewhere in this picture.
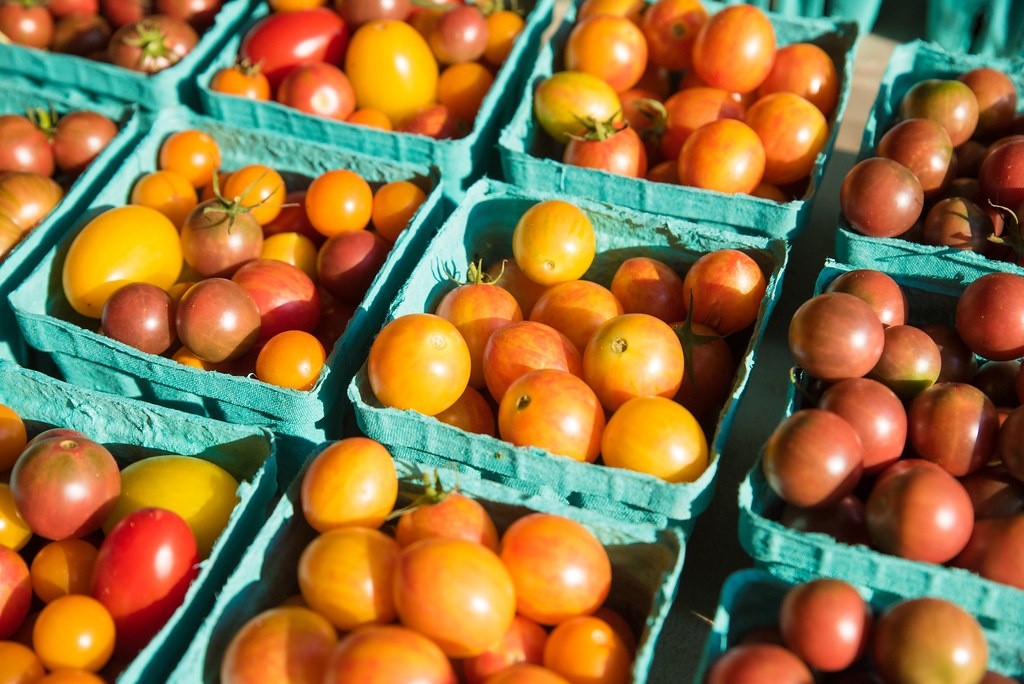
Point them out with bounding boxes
[0,0,1024,684]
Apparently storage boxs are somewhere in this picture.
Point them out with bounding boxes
[0,0,1024,684]
